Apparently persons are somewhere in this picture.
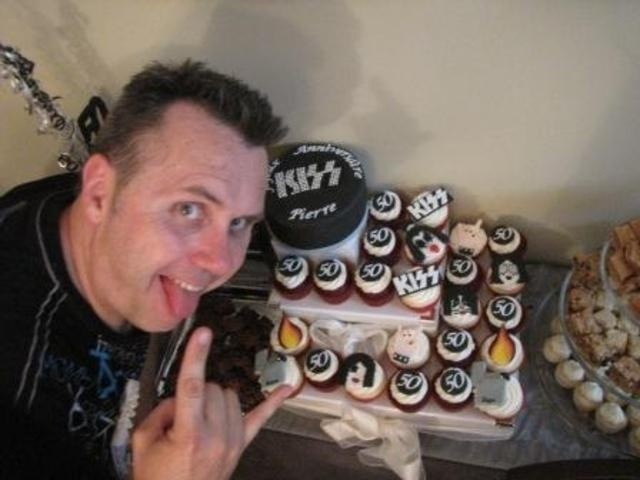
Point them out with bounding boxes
[0,57,294,480]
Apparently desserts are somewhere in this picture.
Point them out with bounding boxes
[257,144,639,451]
